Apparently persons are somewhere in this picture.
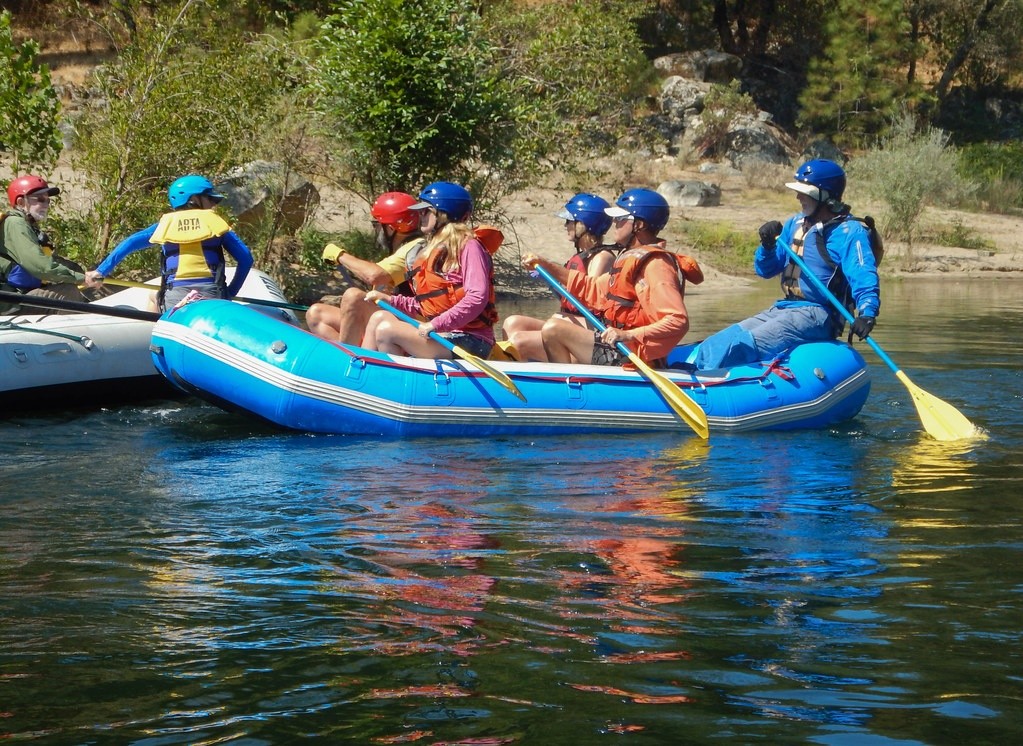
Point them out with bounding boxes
[684,159,881,370]
[500,193,617,366]
[84,175,254,313]
[361,182,498,361]
[0,176,97,316]
[542,188,689,366]
[302,192,422,348]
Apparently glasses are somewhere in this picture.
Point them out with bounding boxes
[565,220,576,225]
[613,214,634,221]
[208,196,219,204]
[420,209,435,217]
[370,221,383,229]
[29,195,50,204]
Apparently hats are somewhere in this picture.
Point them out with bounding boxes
[556,210,575,222]
[205,191,224,204]
[405,202,433,210]
[28,187,60,197]
[785,181,830,202]
[606,206,632,219]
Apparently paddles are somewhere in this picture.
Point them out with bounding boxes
[3,290,162,324]
[775,234,976,442]
[527,252,710,439]
[373,298,527,401]
[92,276,311,312]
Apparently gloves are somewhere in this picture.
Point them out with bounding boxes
[323,244,346,267]
[759,220,783,248]
[852,315,875,341]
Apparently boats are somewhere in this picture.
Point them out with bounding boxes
[149,296,872,435]
[0,266,301,419]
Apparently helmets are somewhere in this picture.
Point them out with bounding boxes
[8,176,48,209]
[616,186,670,233]
[370,191,420,233]
[564,193,613,239]
[168,174,213,208]
[794,159,847,202]
[419,181,473,223]
